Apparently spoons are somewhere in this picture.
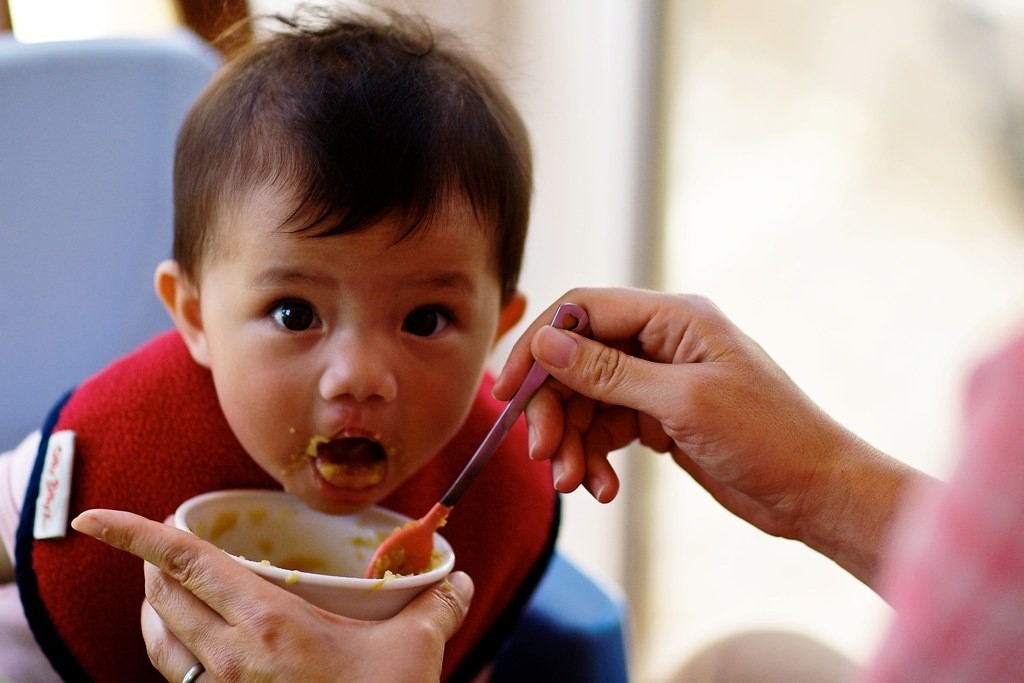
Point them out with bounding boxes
[363,303,589,577]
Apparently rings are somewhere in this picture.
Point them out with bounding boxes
[182,661,205,683]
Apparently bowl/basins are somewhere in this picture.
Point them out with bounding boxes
[174,489,456,620]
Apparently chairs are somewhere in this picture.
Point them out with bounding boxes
[0,35,627,683]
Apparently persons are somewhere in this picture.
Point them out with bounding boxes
[71,288,1024,683]
[0,22,561,683]
